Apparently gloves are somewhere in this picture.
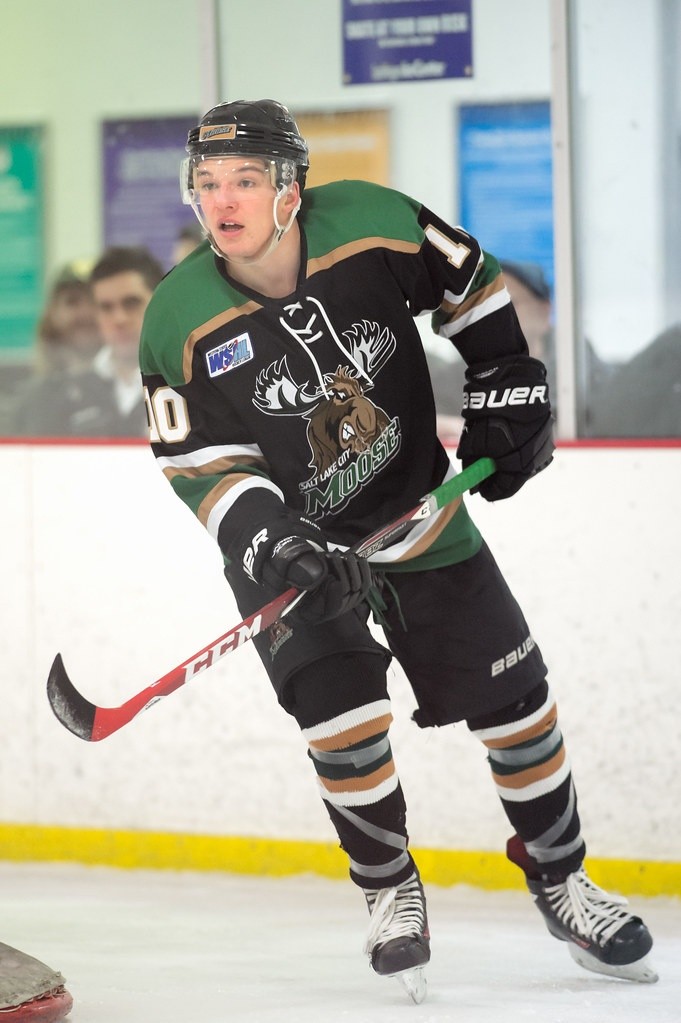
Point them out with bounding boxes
[455,356,557,503]
[236,509,371,625]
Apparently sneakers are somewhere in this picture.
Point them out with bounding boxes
[531,870,659,986]
[360,870,431,1006]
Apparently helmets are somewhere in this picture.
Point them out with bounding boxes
[178,97,310,207]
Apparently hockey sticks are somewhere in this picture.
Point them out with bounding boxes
[44,457,495,744]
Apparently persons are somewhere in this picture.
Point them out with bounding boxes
[139,98,653,976]
[0,225,204,437]
